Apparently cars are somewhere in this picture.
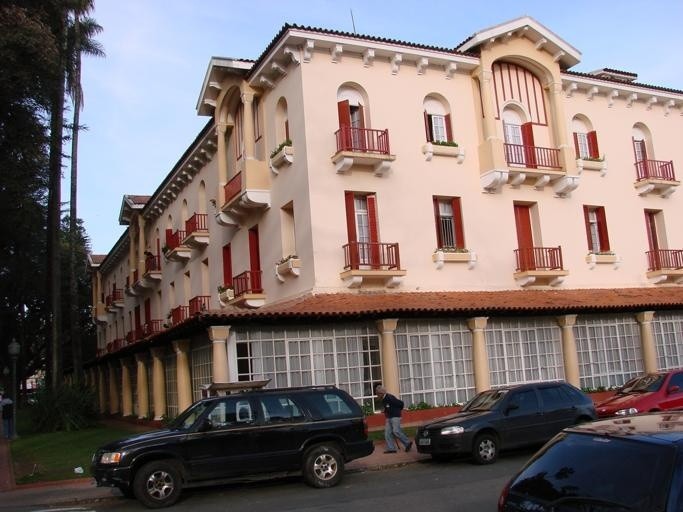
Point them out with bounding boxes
[498,411,683,512]
[595,371,681,417]
[415,380,595,464]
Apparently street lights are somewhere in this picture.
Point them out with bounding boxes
[9,341,21,438]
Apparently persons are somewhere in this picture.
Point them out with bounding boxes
[0,392,12,440]
[375,382,412,456]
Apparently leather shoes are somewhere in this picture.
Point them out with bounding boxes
[384,449,397,454]
[405,441,413,452]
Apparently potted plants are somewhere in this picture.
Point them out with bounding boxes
[217,282,234,302]
[270,140,293,166]
[587,250,616,264]
[431,139,459,156]
[434,247,471,262]
[278,255,301,273]
[161,245,172,256]
[577,154,606,171]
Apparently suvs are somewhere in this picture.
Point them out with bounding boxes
[92,386,375,509]
